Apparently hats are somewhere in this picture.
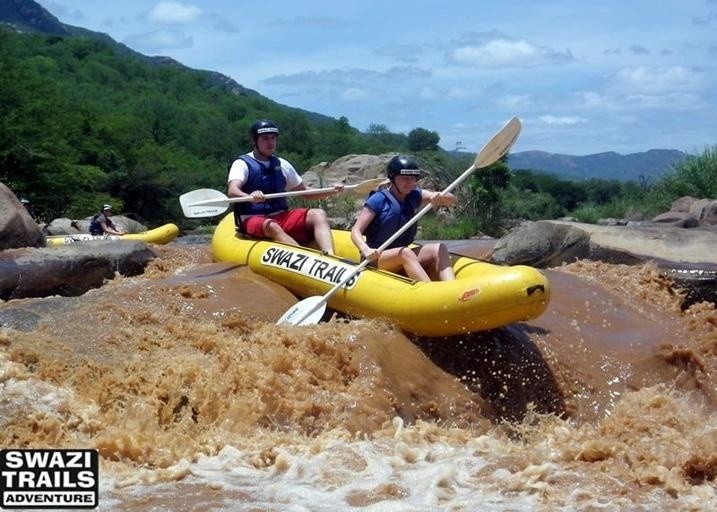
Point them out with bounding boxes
[104,204,112,211]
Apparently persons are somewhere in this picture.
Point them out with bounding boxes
[351,155,456,282]
[228,118,344,255]
[90,204,128,235]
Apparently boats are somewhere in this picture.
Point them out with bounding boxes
[210,211,550,338]
[46,222,179,248]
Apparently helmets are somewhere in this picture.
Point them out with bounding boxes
[387,156,421,178]
[249,119,279,137]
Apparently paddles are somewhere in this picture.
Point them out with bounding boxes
[275,115,522,326]
[179,178,390,218]
